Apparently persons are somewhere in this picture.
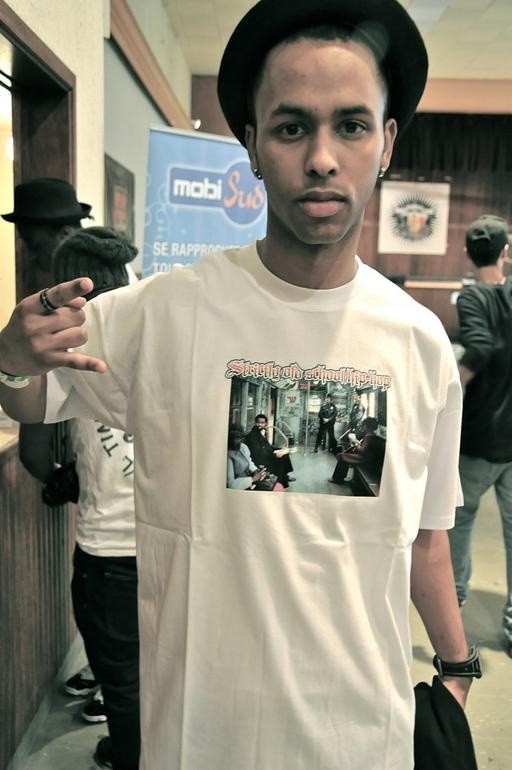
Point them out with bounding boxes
[227,394,378,492]
[445,215,512,658]
[0,178,107,723]
[52,228,138,770]
[0,2,481,770]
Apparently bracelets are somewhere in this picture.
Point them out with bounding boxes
[1,372,33,389]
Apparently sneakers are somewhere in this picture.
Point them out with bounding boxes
[64,658,108,725]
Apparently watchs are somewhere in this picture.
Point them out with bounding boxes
[433,645,482,679]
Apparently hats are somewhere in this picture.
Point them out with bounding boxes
[1,178,92,225]
[466,213,509,271]
[229,422,246,438]
[215,0,429,147]
[52,226,138,295]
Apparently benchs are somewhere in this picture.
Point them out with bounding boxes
[349,431,387,497]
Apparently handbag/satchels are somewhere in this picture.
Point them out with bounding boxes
[252,468,279,490]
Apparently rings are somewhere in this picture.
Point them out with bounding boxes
[40,290,57,312]
